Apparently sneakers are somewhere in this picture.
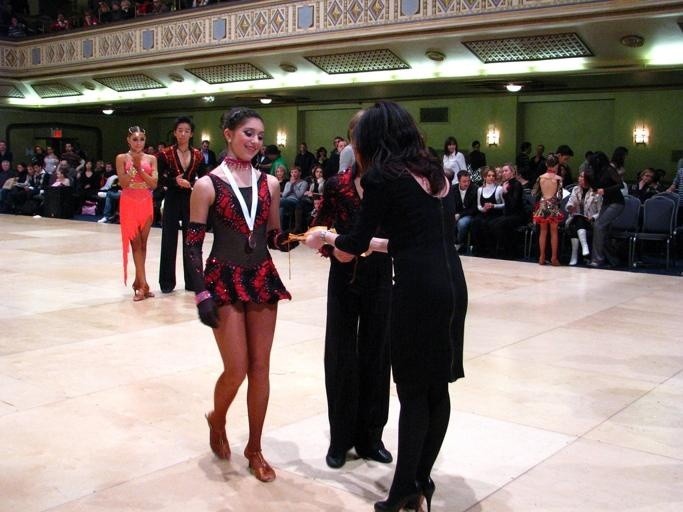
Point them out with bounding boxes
[98,216,110,223]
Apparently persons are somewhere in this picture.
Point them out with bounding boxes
[302,100,468,512]
[1,136,121,224]
[114,125,159,303]
[154,117,205,293]
[181,105,301,483]
[140,136,357,236]
[430,136,683,269]
[311,107,394,468]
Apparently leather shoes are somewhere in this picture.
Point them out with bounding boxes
[369,447,393,462]
[162,288,172,294]
[326,451,346,469]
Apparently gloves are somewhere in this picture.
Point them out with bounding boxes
[195,290,219,328]
[275,231,299,251]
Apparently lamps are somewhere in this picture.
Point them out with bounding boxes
[260,98,273,105]
[503,84,524,92]
[102,107,116,115]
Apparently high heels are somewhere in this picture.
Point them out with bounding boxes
[539,256,547,265]
[204,412,231,460]
[419,476,435,511]
[133,283,149,301]
[551,258,561,265]
[374,480,423,511]
[132,280,155,298]
[243,443,277,482]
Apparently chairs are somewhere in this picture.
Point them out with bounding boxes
[522,189,683,272]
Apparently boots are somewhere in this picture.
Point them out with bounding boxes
[577,228,590,256]
[570,238,579,266]
